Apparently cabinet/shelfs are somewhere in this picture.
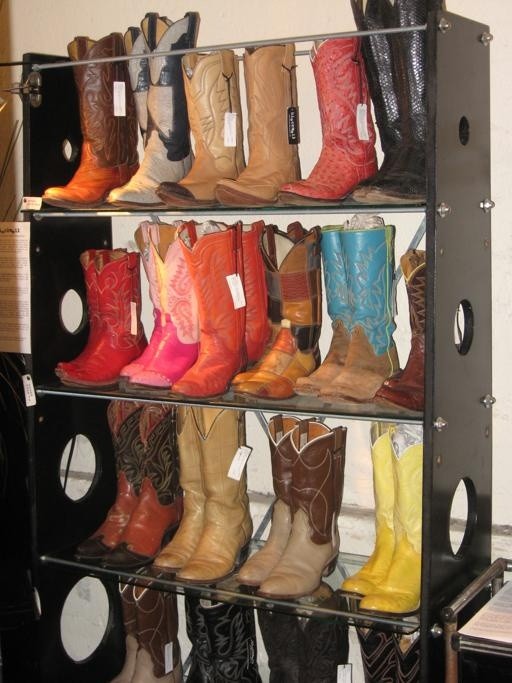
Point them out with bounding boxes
[22,11,491,682]
